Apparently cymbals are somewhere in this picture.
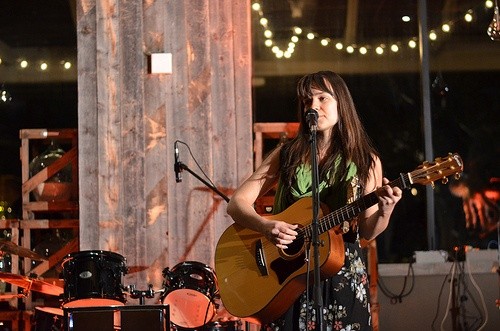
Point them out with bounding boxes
[0,272,64,296]
[125,265,149,275]
[0,238,47,262]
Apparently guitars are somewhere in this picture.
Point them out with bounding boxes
[213,151,464,322]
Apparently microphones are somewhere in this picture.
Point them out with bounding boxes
[174,144,182,183]
[305,108,318,128]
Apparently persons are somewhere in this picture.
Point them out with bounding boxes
[227,71,402,331]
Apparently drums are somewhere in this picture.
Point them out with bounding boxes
[34,307,71,331]
[61,250,127,307]
[160,260,219,328]
